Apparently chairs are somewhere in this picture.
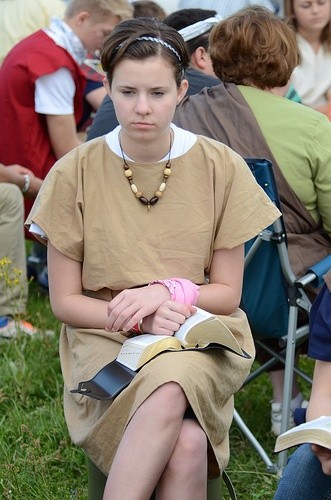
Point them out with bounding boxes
[231,156,331,490]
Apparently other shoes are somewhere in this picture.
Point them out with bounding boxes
[28,255,49,287]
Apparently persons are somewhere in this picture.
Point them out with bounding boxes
[0,0,331,436]
[24,18,282,500]
[274,268,331,500]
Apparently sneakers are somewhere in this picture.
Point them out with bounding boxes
[0,318,55,340]
[270,400,309,436]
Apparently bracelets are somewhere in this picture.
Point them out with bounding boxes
[130,319,143,333]
[23,174,30,194]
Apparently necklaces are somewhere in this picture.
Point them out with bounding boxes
[119,133,171,211]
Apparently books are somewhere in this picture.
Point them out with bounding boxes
[273,416,331,454]
[69,305,253,401]
